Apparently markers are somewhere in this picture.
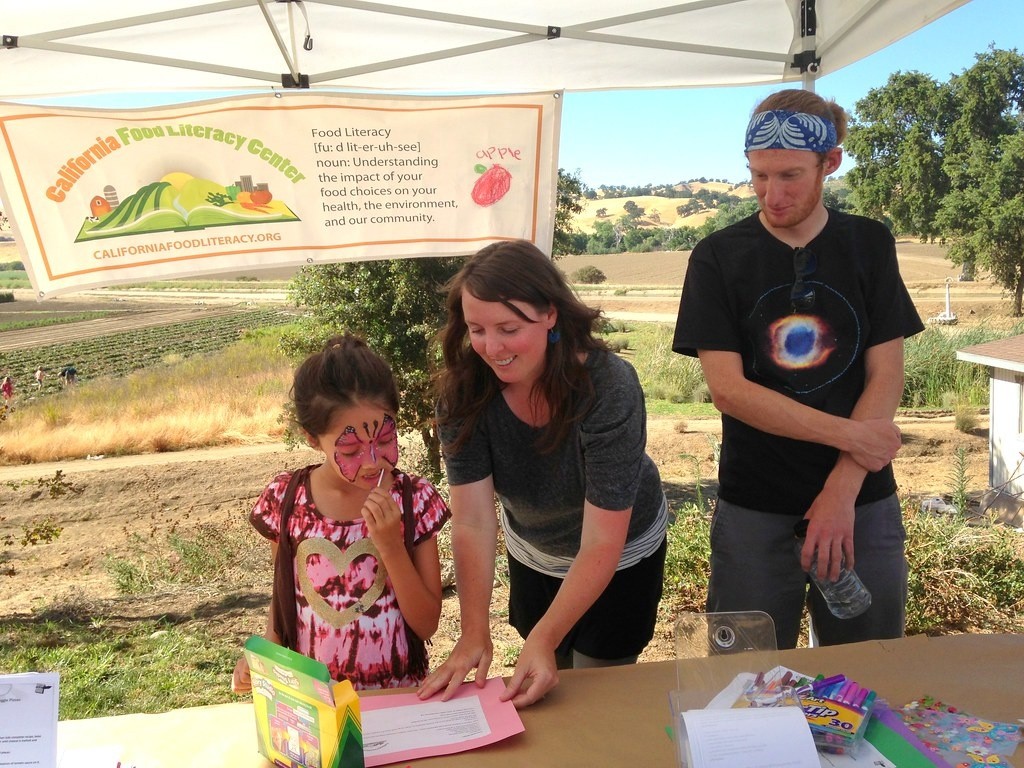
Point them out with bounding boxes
[755,671,877,756]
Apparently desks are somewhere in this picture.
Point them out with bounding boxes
[58,633,1024,767]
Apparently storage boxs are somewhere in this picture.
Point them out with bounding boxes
[244,634,365,767]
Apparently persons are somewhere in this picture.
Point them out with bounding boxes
[671,89,926,655]
[58,367,76,385]
[36,366,44,391]
[1,377,13,403]
[416,239,668,707]
[230,330,453,694]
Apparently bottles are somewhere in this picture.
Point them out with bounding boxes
[794,519,871,618]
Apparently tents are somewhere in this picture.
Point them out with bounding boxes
[0,0,975,95]
